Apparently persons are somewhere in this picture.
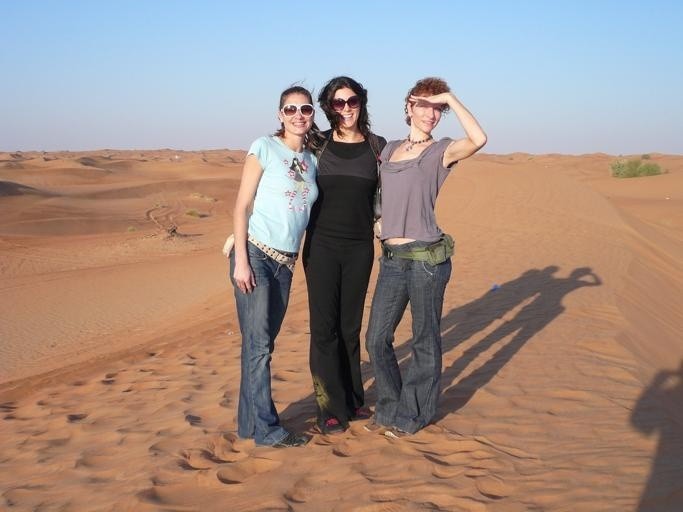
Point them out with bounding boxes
[362,77,487,437]
[305,76,389,434]
[222,86,319,446]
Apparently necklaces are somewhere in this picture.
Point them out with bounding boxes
[405,135,433,151]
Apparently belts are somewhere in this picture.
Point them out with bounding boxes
[246,235,298,275]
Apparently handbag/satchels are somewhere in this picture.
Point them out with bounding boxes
[425,234,454,265]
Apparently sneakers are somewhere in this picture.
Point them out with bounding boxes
[347,407,370,421]
[363,420,384,431]
[272,432,307,447]
[322,417,344,434]
[384,425,408,438]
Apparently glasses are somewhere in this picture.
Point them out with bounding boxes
[330,95,361,111]
[280,103,314,118]
